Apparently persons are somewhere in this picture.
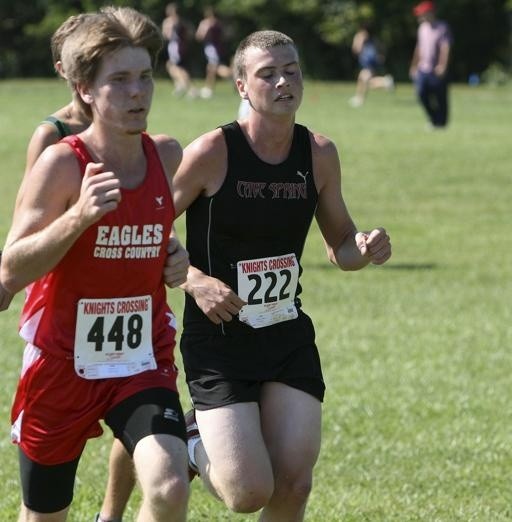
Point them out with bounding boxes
[17,13,138,522]
[161,3,191,99]
[1,4,192,522]
[345,26,395,111]
[408,0,453,134]
[195,5,233,99]
[165,29,391,522]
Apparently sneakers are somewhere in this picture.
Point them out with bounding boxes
[181,409,199,483]
